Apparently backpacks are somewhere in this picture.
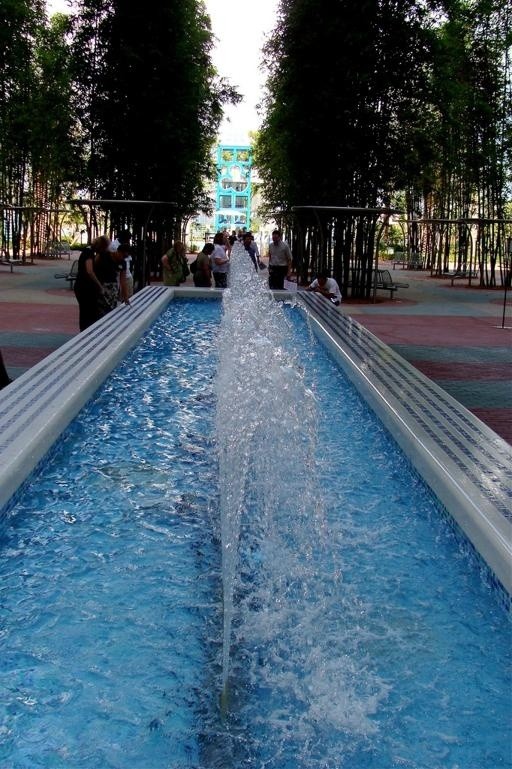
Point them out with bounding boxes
[175,259,190,283]
[190,256,198,274]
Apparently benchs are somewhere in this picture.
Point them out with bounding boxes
[348,268,409,299]
[55,259,78,290]
[442,263,480,278]
[45,240,70,260]
[0,249,21,273]
[391,252,423,270]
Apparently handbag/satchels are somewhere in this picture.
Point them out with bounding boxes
[258,261,267,271]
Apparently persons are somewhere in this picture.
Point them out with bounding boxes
[267,230,293,290]
[216,226,267,276]
[94,242,131,307]
[161,240,190,287]
[192,242,216,287]
[72,236,110,334]
[305,270,343,307]
[105,230,135,300]
[211,232,229,287]
